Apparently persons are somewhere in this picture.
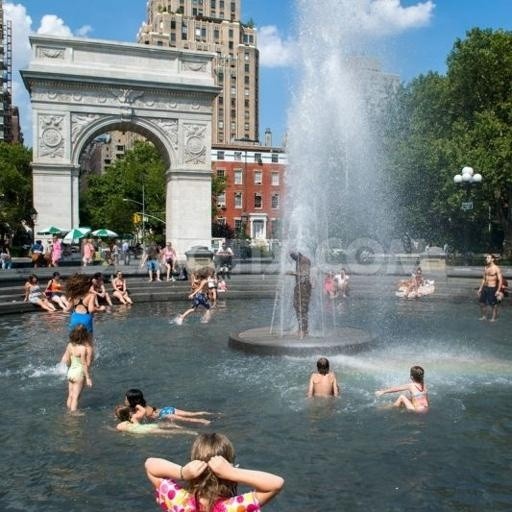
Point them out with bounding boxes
[124,388,213,427]
[29,235,130,267]
[0,245,13,270]
[115,404,199,438]
[397,255,507,324]
[142,239,236,326]
[287,249,351,340]
[22,270,134,418]
[308,358,340,398]
[374,366,430,415]
[146,433,284,512]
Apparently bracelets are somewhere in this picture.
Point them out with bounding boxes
[180,466,184,481]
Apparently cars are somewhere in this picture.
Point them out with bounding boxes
[115,233,136,245]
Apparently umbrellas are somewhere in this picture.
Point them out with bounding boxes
[35,225,120,244]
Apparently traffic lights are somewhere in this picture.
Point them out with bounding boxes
[137,214,142,222]
[133,213,137,223]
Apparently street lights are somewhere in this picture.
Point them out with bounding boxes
[453,165,484,266]
[123,199,144,238]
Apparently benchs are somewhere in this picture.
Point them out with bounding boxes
[10,255,33,269]
